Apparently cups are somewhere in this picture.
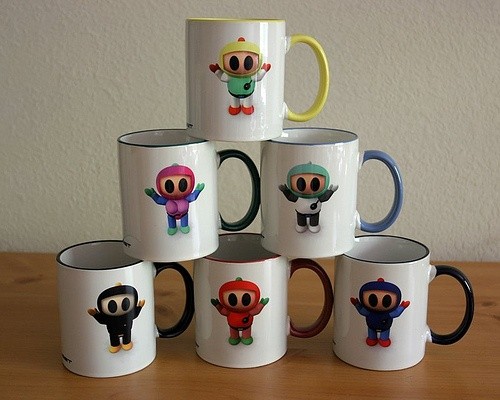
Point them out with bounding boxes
[56,240,194,377]
[334,235,474,371]
[194,233,334,368]
[260,128,403,258]
[117,129,261,262]
[186,18,329,142]
